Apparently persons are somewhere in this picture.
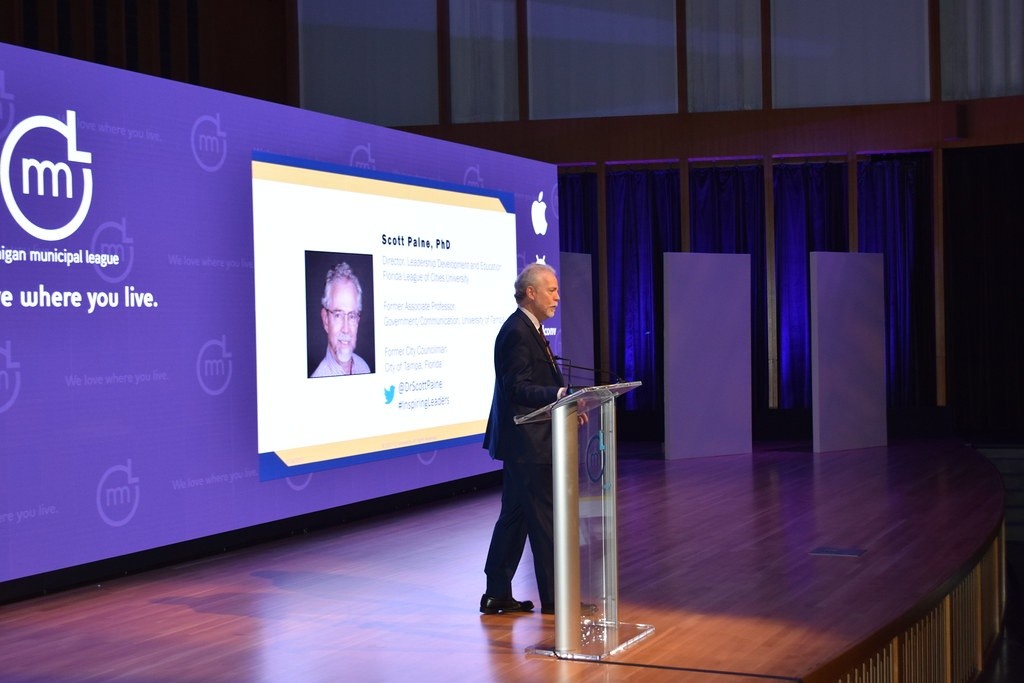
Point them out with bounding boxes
[307,261,370,379]
[478,262,599,617]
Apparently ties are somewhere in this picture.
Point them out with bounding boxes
[539,325,557,372]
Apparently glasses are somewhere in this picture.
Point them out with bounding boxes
[325,308,358,323]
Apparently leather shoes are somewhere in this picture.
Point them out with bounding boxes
[540,600,598,615]
[479,592,535,614]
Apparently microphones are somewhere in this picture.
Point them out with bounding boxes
[544,356,625,396]
